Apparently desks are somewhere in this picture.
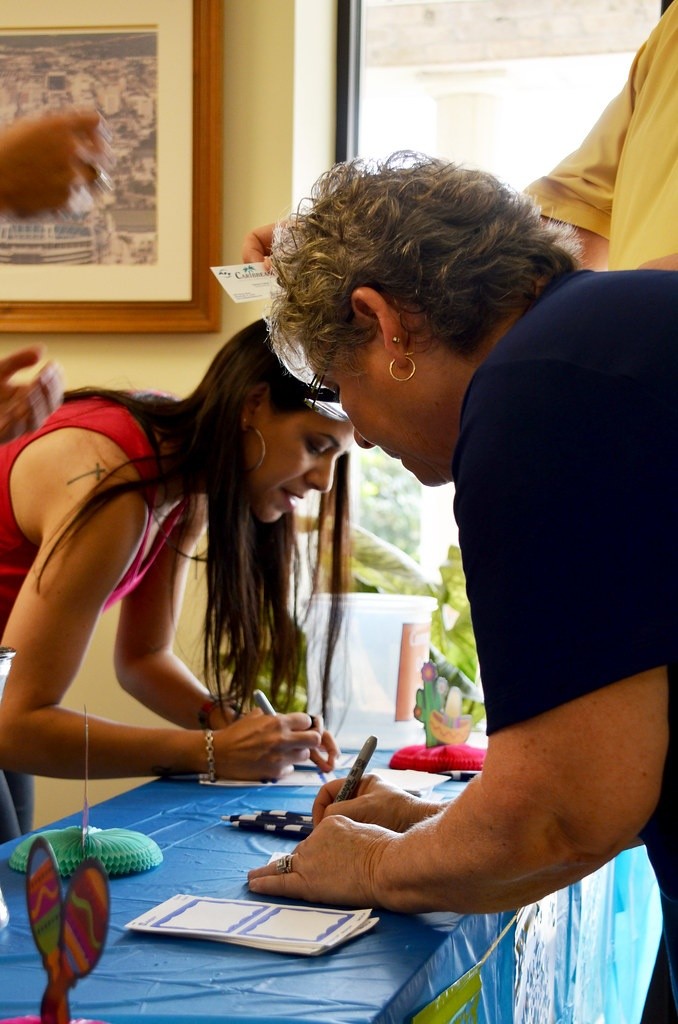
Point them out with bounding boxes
[0,748,662,1024]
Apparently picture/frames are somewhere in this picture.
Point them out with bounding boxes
[0,0,225,334]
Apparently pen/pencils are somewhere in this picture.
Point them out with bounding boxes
[445,770,478,783]
[253,688,276,717]
[334,735,377,803]
[220,809,313,841]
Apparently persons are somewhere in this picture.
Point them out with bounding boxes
[245,159,678,1024]
[513,1,678,271]
[0,105,104,449]
[0,315,357,844]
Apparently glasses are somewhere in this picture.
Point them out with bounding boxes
[304,282,385,422]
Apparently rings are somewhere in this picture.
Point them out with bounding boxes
[276,854,295,874]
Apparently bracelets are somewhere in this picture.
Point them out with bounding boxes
[197,696,217,730]
[205,729,218,782]
